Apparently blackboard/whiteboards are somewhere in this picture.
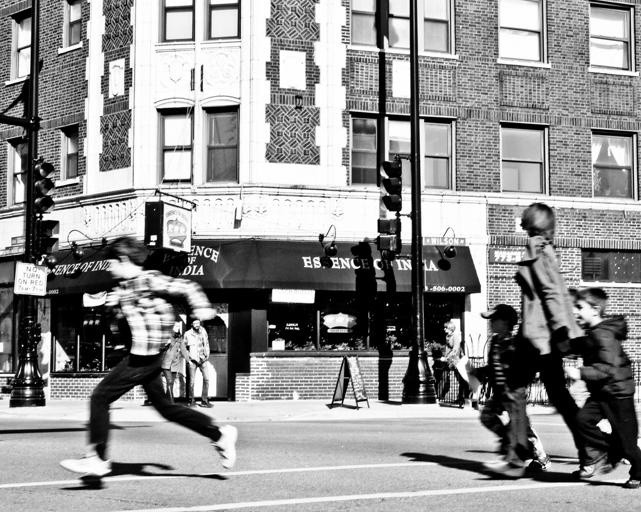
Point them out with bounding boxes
[346,353,371,401]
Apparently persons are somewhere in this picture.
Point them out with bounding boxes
[59,237,237,478]
[556,288,641,488]
[161,321,193,404]
[467,304,551,472]
[437,321,466,404]
[183,318,214,408]
[484,204,609,479]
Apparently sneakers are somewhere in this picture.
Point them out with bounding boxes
[622,478,641,490]
[210,424,238,470]
[482,438,631,480]
[182,401,215,409]
[58,452,112,477]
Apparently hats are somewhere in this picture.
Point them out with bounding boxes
[480,304,518,323]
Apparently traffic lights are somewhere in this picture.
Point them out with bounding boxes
[373,218,401,256]
[29,218,59,258]
[29,159,57,221]
[378,154,403,214]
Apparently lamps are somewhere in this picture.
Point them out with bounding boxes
[67,229,94,261]
[319,224,340,257]
[443,226,457,258]
